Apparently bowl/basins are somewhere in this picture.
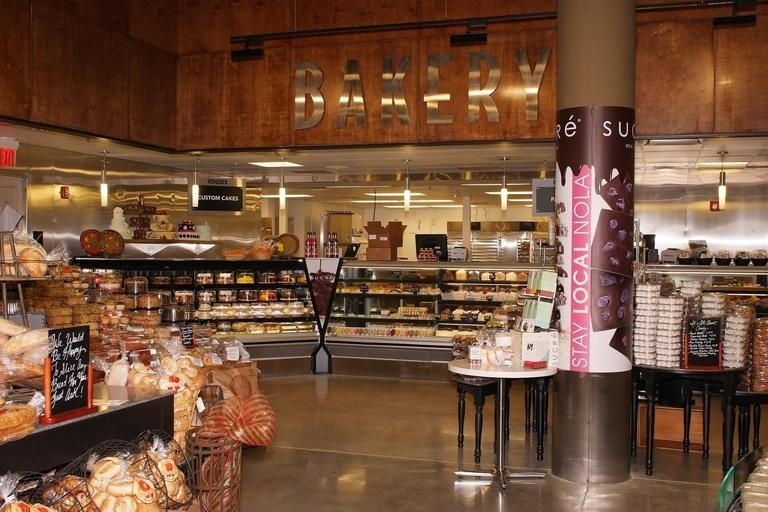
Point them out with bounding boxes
[679,257,768,266]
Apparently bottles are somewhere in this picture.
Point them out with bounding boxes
[305,232,339,258]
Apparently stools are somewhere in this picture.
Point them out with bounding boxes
[720,388,768,460]
[451,373,511,463]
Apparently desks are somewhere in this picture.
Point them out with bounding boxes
[633,364,751,478]
[446,358,557,488]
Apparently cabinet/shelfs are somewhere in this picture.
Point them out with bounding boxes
[642,262,768,319]
[74,254,556,351]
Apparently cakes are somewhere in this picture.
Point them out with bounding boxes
[123,273,307,320]
[440,271,527,322]
[108,206,200,241]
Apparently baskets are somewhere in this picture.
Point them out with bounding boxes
[185,427,241,511]
[0,431,195,512]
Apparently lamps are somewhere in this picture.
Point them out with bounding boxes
[98,148,112,207]
[716,151,729,210]
[191,156,288,209]
[402,156,509,211]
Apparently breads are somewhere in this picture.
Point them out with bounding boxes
[0,244,252,512]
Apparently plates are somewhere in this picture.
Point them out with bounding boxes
[80,228,124,257]
[273,233,299,257]
